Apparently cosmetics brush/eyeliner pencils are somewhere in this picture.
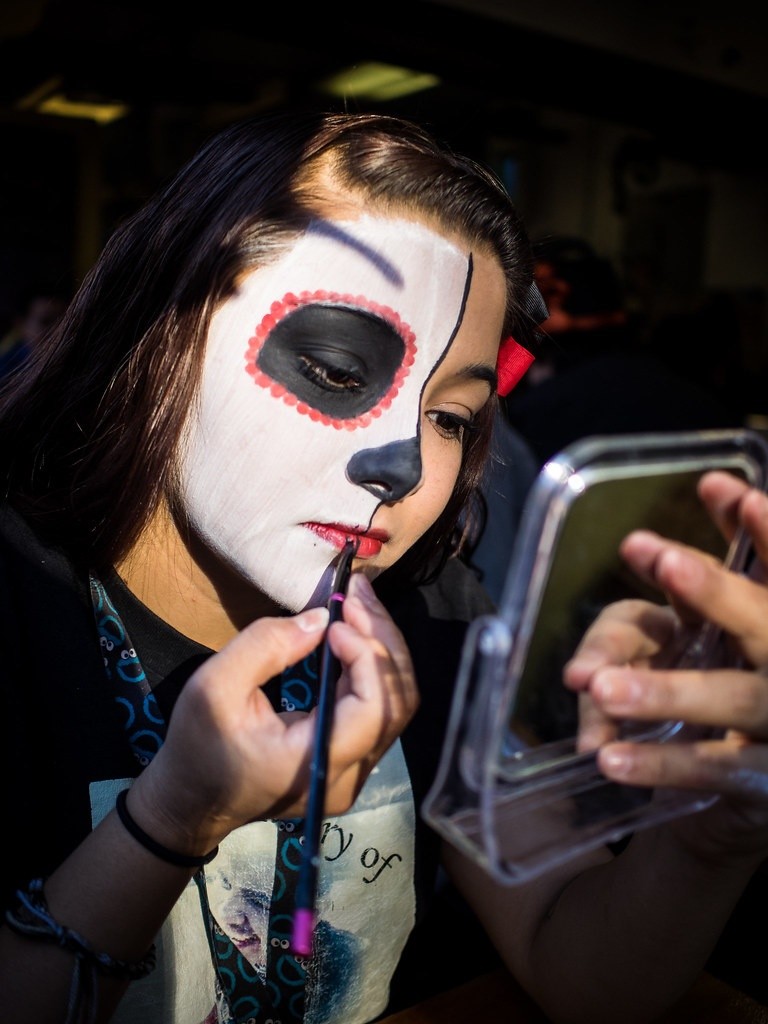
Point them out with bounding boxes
[291,540,353,960]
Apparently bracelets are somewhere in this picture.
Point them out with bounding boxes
[117,789,220,867]
[6,875,158,1024]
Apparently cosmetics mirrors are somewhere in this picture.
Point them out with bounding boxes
[423,428,766,887]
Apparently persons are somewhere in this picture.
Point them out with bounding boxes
[2,104,768,1024]
[562,470,752,753]
